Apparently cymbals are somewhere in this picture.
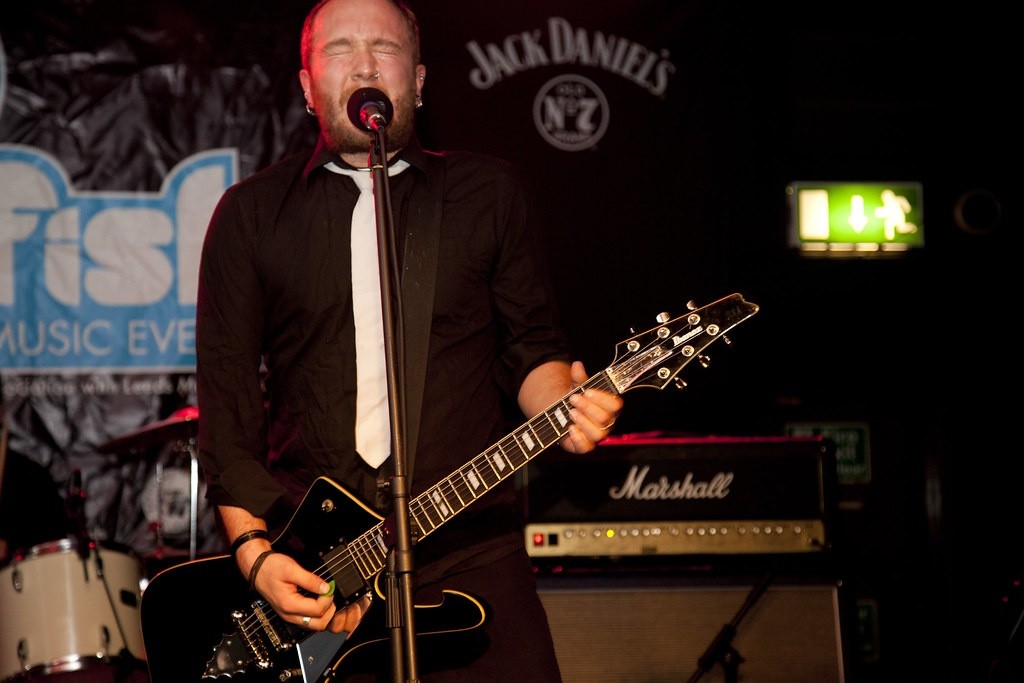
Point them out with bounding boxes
[95,404,199,447]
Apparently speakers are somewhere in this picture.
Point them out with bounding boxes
[526,553,865,683]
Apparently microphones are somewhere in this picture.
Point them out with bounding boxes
[65,470,91,561]
[347,87,394,132]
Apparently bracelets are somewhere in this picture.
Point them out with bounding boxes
[231,530,270,555]
[247,550,275,589]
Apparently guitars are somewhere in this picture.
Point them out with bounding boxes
[140,289,759,683]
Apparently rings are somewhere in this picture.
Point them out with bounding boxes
[600,421,615,430]
[302,616,311,625]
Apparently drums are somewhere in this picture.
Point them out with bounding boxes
[0,539,153,683]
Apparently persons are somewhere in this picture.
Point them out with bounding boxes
[195,0,623,683]
[0,446,69,570]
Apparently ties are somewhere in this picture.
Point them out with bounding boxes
[323,160,411,470]
